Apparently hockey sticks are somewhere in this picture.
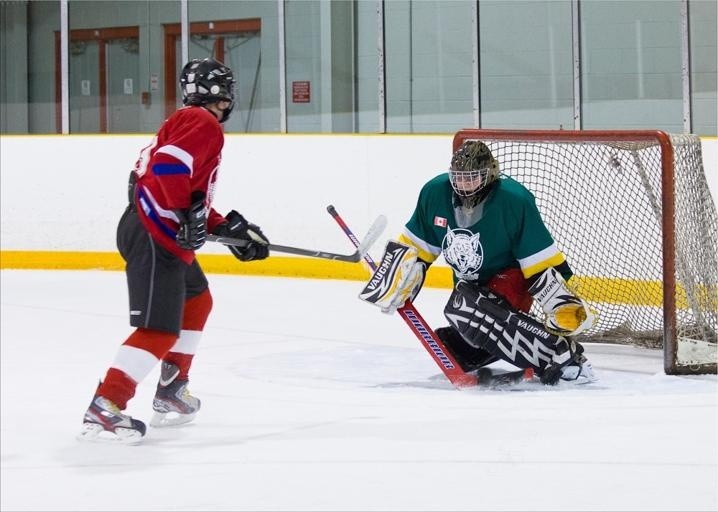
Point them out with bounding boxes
[326,206,534,391]
[205,215,388,263]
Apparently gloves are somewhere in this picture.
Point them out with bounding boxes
[176,190,210,251]
[216,208,271,263]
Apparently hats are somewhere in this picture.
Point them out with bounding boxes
[178,56,236,106]
[449,139,501,198]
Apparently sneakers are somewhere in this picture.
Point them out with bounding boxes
[557,355,597,383]
[83,389,146,438]
[152,360,201,415]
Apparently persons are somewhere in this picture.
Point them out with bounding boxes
[83,56,269,437]
[358,140,593,384]
